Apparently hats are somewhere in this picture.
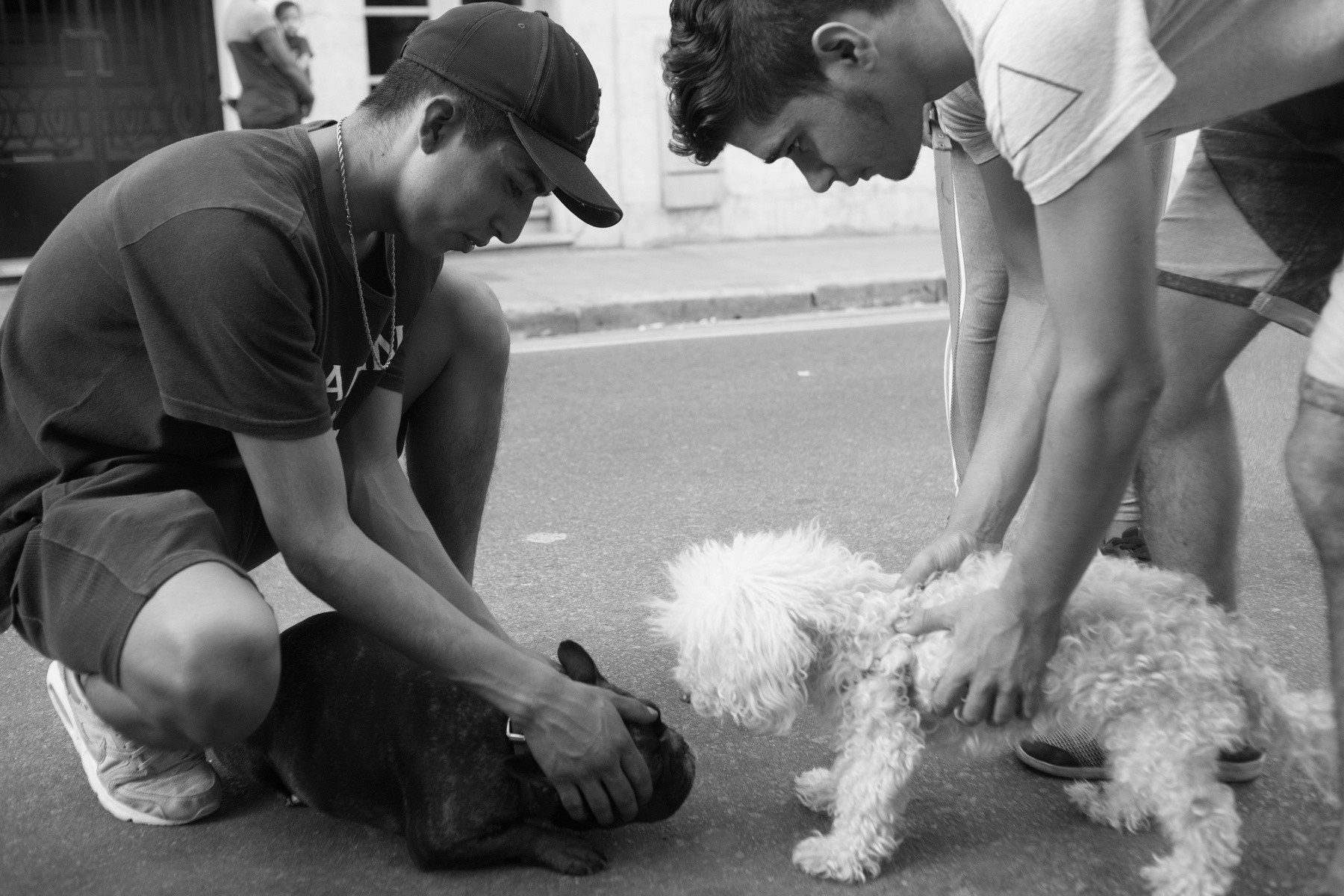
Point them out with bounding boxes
[400,2,622,229]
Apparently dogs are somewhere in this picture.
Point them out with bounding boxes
[194,603,699,879]
[631,506,1339,896]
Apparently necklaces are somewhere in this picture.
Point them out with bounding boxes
[337,117,395,369]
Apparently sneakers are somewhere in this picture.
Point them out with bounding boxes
[44,661,223,826]
[1016,717,1268,781]
[1100,527,1153,567]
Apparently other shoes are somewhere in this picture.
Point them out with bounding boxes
[1112,497,1139,521]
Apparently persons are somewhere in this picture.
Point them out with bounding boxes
[228,1,313,110]
[225,0,314,130]
[660,0,1344,895]
[0,2,656,822]
